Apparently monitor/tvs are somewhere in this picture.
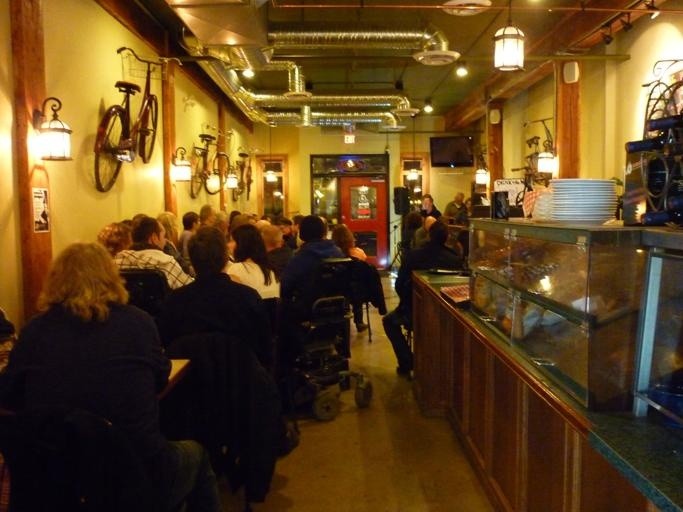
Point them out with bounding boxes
[429,135,474,168]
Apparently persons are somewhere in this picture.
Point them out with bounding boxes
[331,225,367,332]
[179,205,306,274]
[220,223,282,299]
[98,212,195,289]
[383,193,473,374]
[154,227,276,508]
[279,216,345,300]
[1,241,221,512]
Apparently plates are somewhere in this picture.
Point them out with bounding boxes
[531,178,619,225]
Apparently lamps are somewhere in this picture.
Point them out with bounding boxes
[171,133,238,201]
[492,1,529,70]
[470,143,491,186]
[524,134,558,175]
[34,95,80,172]
[420,55,469,117]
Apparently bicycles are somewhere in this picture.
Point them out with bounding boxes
[230,142,264,202]
[93,45,184,193]
[511,115,555,204]
[189,125,231,199]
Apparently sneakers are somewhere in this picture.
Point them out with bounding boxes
[355,323,369,333]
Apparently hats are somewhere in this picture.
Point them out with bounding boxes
[299,216,325,242]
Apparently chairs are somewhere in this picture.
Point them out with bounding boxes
[1,256,373,511]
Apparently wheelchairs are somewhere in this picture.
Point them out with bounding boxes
[277,257,372,422]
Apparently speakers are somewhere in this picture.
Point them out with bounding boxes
[394,187,411,216]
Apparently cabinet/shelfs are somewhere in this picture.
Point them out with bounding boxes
[465,216,683,413]
